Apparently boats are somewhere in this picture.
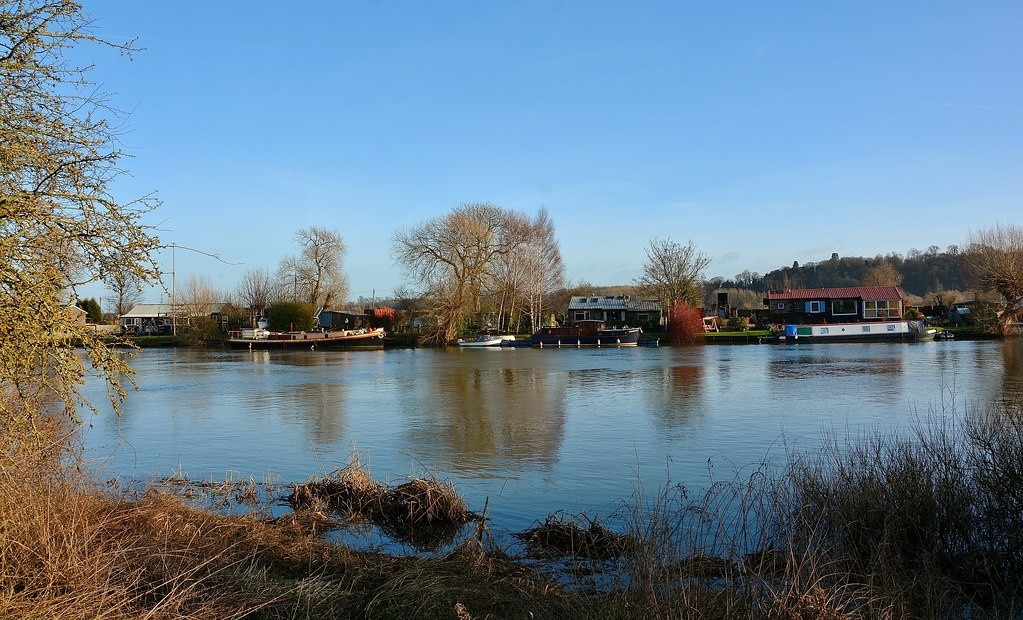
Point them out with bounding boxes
[457,335,504,349]
[224,323,388,352]
[529,320,641,348]
